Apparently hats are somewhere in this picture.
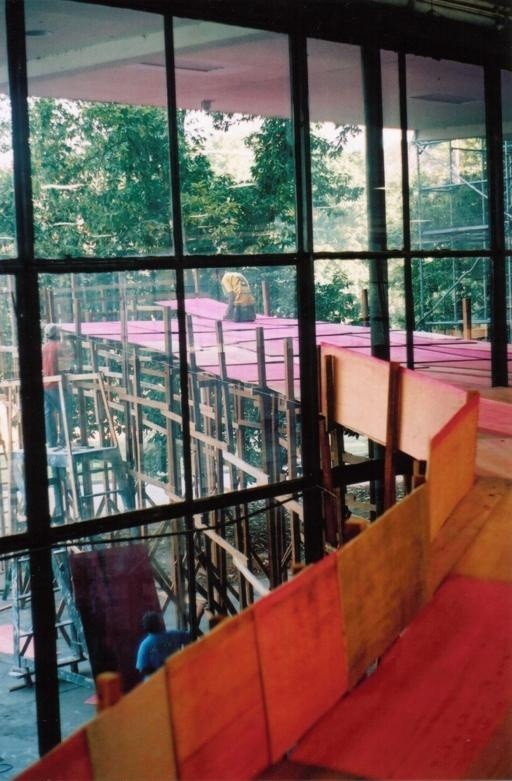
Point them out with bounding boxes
[44,323,57,339]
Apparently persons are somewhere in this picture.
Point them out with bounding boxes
[136,602,208,682]
[211,271,256,322]
[41,324,74,451]
